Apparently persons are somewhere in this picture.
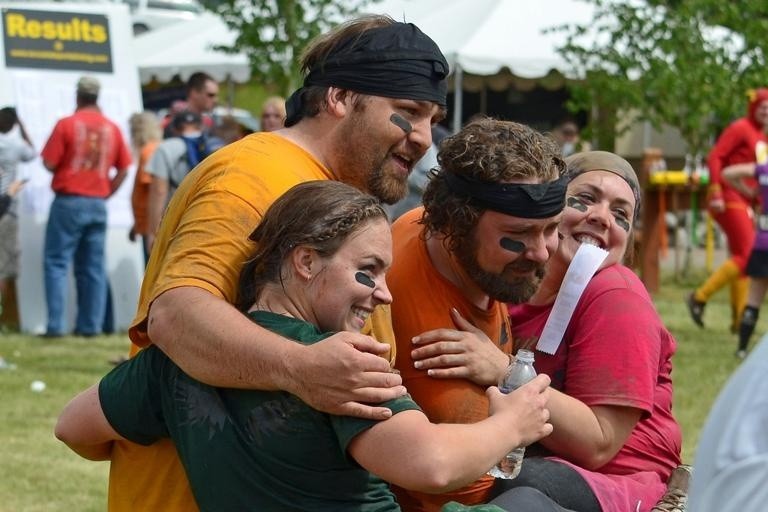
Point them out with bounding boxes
[681,87,768,335]
[548,120,594,157]
[128,110,163,271]
[40,74,134,338]
[721,160,767,361]
[387,120,569,512]
[146,107,230,259]
[105,14,452,512]
[410,152,686,512]
[162,71,220,133]
[261,95,289,130]
[0,177,32,232]
[0,107,38,333]
[55,181,553,512]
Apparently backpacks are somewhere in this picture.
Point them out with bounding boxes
[176,132,227,171]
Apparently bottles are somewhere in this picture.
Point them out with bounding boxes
[485,349,537,480]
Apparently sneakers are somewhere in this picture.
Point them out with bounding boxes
[685,292,707,327]
[729,323,740,332]
[735,348,746,362]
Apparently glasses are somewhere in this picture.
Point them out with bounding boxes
[206,91,218,99]
[262,113,282,119]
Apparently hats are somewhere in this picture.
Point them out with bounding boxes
[77,77,101,95]
[173,110,203,126]
